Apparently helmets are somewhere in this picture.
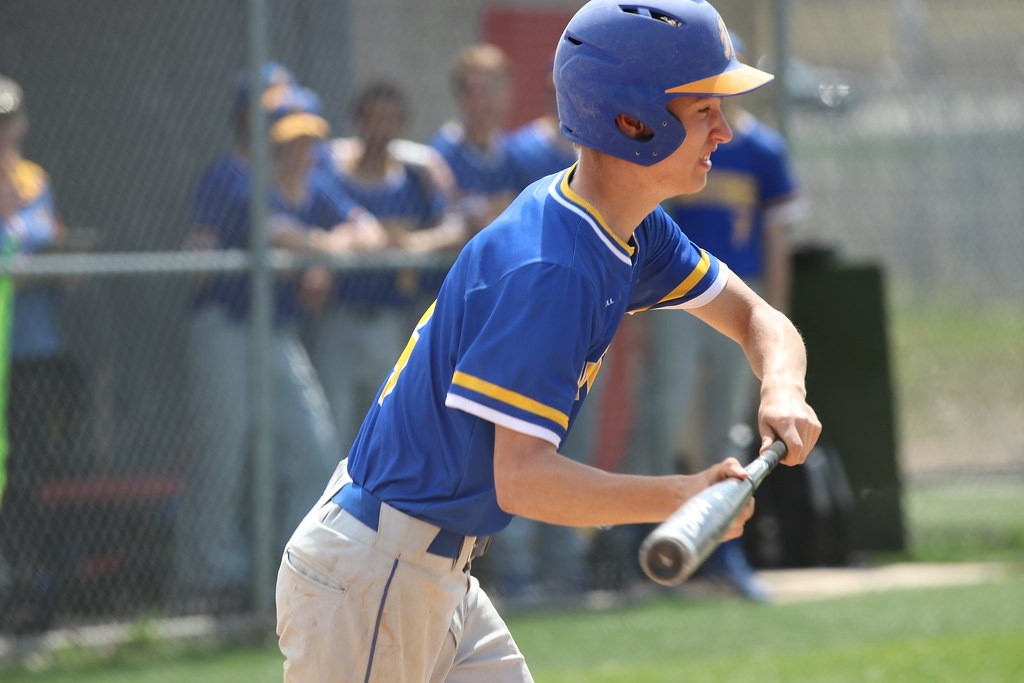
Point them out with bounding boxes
[553,0,775,167]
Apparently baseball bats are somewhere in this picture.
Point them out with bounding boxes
[635,435,790,591]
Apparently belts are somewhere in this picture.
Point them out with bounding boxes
[331,483,493,560]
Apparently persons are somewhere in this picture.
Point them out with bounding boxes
[170,33,796,610]
[274,0,823,683]
[0,78,79,633]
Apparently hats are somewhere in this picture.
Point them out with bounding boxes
[257,85,333,147]
[0,74,24,114]
[233,59,296,117]
